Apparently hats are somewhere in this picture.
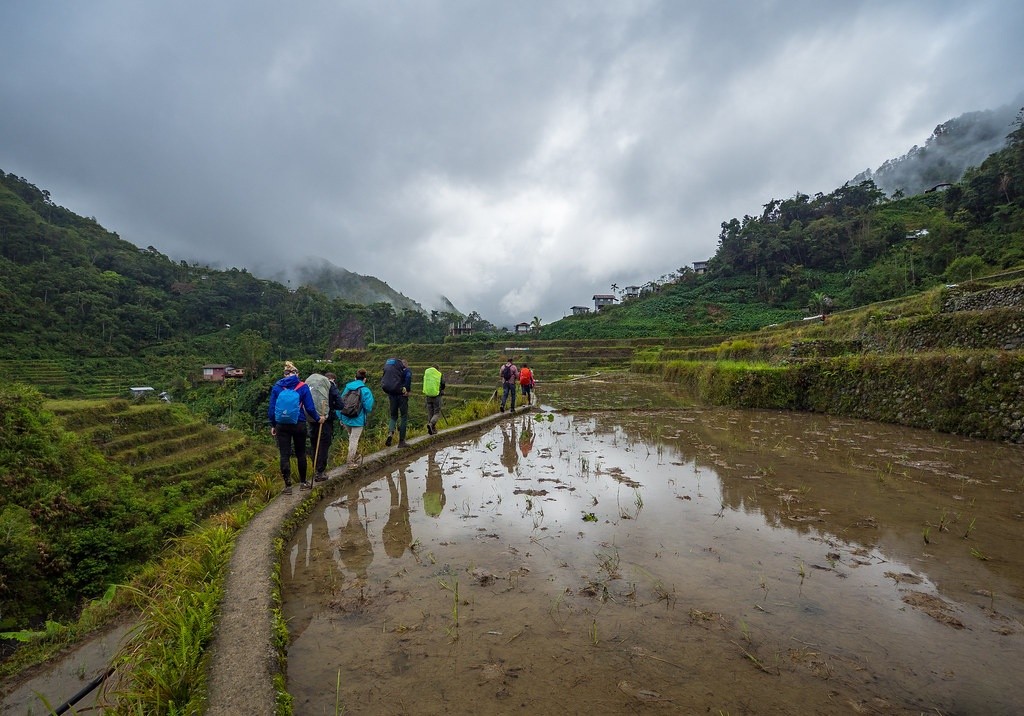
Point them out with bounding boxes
[431,363,440,371]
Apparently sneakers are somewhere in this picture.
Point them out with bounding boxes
[281,487,292,495]
[299,481,311,491]
[346,463,358,469]
[427,423,437,435]
[315,473,329,482]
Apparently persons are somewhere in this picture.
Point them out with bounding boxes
[380,359,412,448]
[269,361,325,495]
[500,358,534,412]
[336,368,375,464]
[304,372,344,482]
[424,363,446,435]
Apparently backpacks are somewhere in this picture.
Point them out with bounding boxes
[422,367,442,397]
[520,367,531,385]
[381,358,406,396]
[503,364,514,381]
[275,382,306,425]
[339,386,367,419]
[304,373,336,422]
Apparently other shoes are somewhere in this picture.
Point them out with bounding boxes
[397,441,410,448]
[522,404,525,407]
[385,434,392,447]
[528,403,532,405]
[500,405,505,413]
[510,409,516,412]
